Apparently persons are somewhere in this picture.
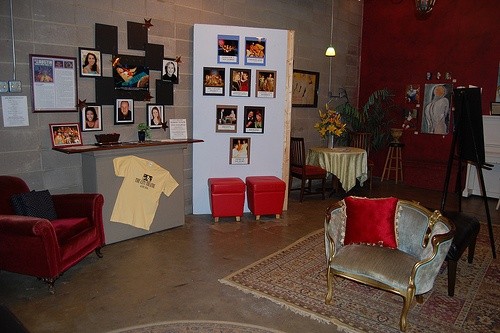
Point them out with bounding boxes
[206,73,222,85]
[56,62,62,68]
[233,140,248,157]
[54,127,78,145]
[233,72,248,86]
[250,42,264,57]
[220,44,234,53]
[83,53,98,74]
[218,109,235,124]
[118,101,131,121]
[246,110,262,127]
[38,70,52,82]
[85,107,99,129]
[151,107,161,124]
[163,62,176,80]
[259,74,274,91]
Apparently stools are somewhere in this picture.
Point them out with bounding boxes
[380,143,404,184]
[208,176,286,222]
[426,207,481,297]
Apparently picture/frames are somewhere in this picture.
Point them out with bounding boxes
[29,47,179,149]
[292,69,320,108]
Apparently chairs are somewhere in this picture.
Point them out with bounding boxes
[0,175,105,296]
[288,137,327,202]
[322,196,455,332]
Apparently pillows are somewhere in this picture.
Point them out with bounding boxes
[338,196,400,250]
[11,189,58,222]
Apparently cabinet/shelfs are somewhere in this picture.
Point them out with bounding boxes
[52,138,204,246]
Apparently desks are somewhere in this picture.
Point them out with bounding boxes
[305,148,368,198]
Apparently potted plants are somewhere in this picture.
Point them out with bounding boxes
[137,122,152,141]
[328,86,397,155]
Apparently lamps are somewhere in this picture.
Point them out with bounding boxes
[325,0,335,57]
[415,0,436,15]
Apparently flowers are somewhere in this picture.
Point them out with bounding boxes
[314,108,347,138]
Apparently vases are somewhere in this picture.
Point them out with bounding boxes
[327,134,334,149]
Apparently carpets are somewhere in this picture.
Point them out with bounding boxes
[103,319,290,333]
[217,221,500,333]
